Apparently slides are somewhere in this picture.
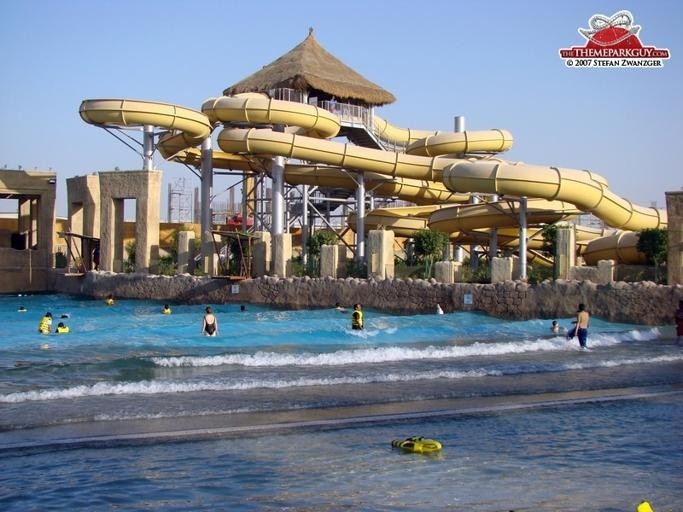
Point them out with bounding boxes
[78,93,667,268]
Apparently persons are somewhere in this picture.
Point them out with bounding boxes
[18,307,27,312]
[674,300,683,343]
[352,304,364,330]
[162,304,171,314]
[106,294,114,305]
[39,312,71,334]
[335,303,342,309]
[202,307,218,337]
[550,303,589,349]
[240,306,245,311]
